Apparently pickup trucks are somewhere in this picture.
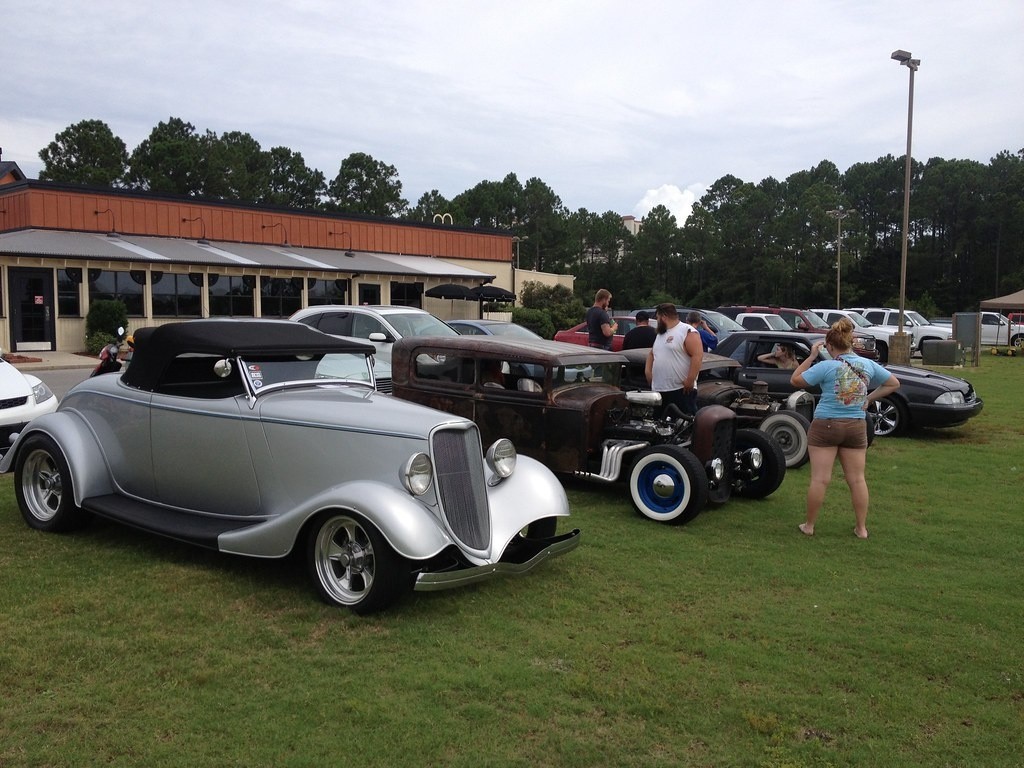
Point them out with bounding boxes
[931,311,1024,347]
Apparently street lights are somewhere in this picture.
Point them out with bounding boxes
[890,49,921,333]
[826,210,842,310]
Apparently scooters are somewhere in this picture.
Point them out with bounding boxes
[89,326,134,380]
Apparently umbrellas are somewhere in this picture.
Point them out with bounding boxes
[425,283,516,320]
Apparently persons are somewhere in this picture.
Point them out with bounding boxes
[686,311,718,381]
[760,343,798,370]
[585,290,618,350]
[791,318,900,538]
[646,304,702,417]
[623,311,657,351]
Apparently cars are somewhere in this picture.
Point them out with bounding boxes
[0,357,60,465]
[707,331,984,436]
[287,303,466,368]
[391,336,788,525]
[588,348,810,468]
[806,308,916,363]
[411,318,592,381]
[554,316,663,355]
[1008,312,1024,326]
[623,303,881,368]
[0,318,582,617]
[313,351,394,401]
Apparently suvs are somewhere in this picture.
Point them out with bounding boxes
[843,307,956,358]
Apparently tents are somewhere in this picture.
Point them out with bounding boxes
[980,288,1024,354]
